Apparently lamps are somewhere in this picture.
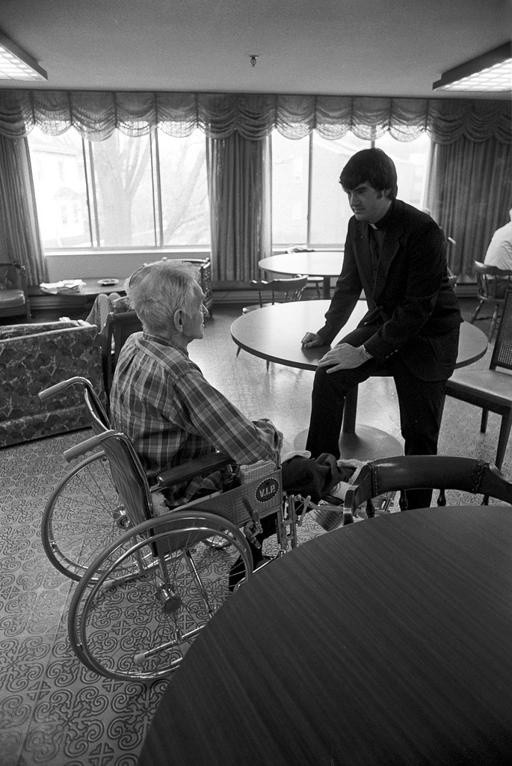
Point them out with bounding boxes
[0,33,48,82]
[432,42,512,91]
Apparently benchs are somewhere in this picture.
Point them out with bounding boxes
[29,281,268,310]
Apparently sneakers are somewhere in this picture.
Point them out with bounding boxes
[228,555,275,592]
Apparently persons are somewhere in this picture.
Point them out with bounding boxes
[300,147,462,513]
[481,206,512,299]
[108,262,324,590]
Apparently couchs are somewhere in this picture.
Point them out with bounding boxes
[0,315,105,449]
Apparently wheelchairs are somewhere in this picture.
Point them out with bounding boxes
[38,376,396,681]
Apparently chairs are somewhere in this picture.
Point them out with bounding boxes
[471,260,512,341]
[447,291,512,471]
[108,310,141,385]
[341,454,512,523]
[0,262,30,322]
[235,275,312,373]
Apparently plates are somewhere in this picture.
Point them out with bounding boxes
[99,278,117,286]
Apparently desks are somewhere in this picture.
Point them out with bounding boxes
[137,505,512,766]
[258,251,345,299]
[59,278,128,296]
[230,298,488,466]
